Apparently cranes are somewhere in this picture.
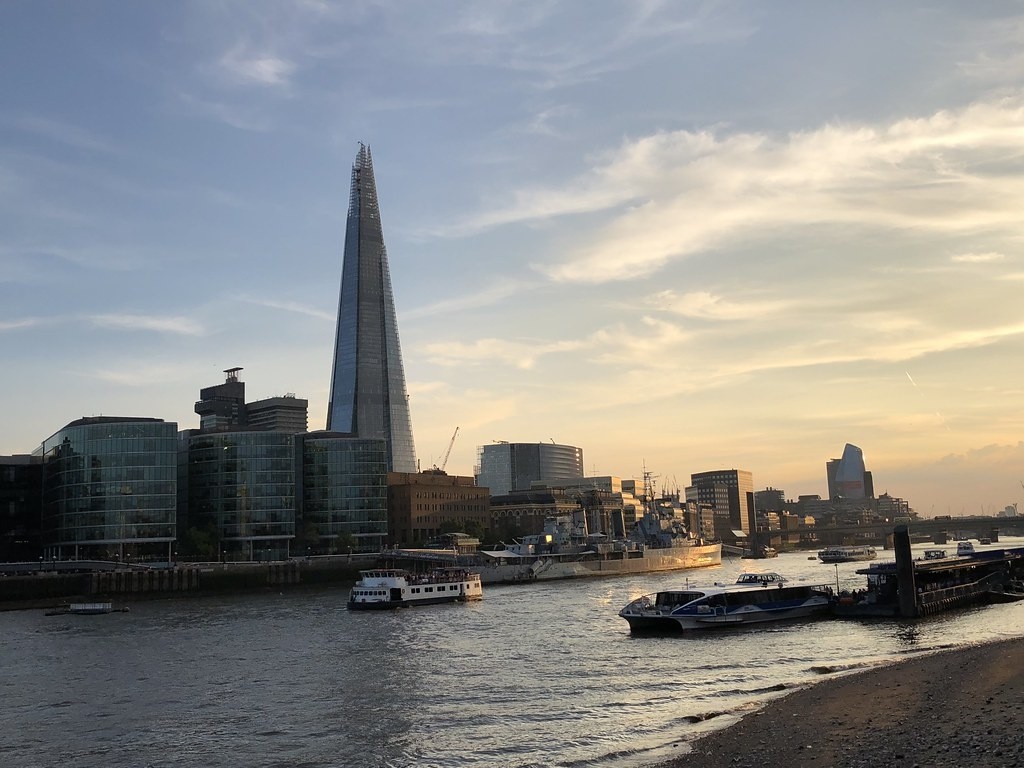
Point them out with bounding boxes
[433,426,459,471]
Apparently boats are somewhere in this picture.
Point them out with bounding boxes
[952,537,968,541]
[379,507,723,585]
[44,600,130,616]
[979,538,992,545]
[818,547,877,563]
[741,546,778,559]
[714,572,789,587]
[618,580,838,634]
[923,550,948,560]
[344,568,484,610]
[957,541,975,556]
